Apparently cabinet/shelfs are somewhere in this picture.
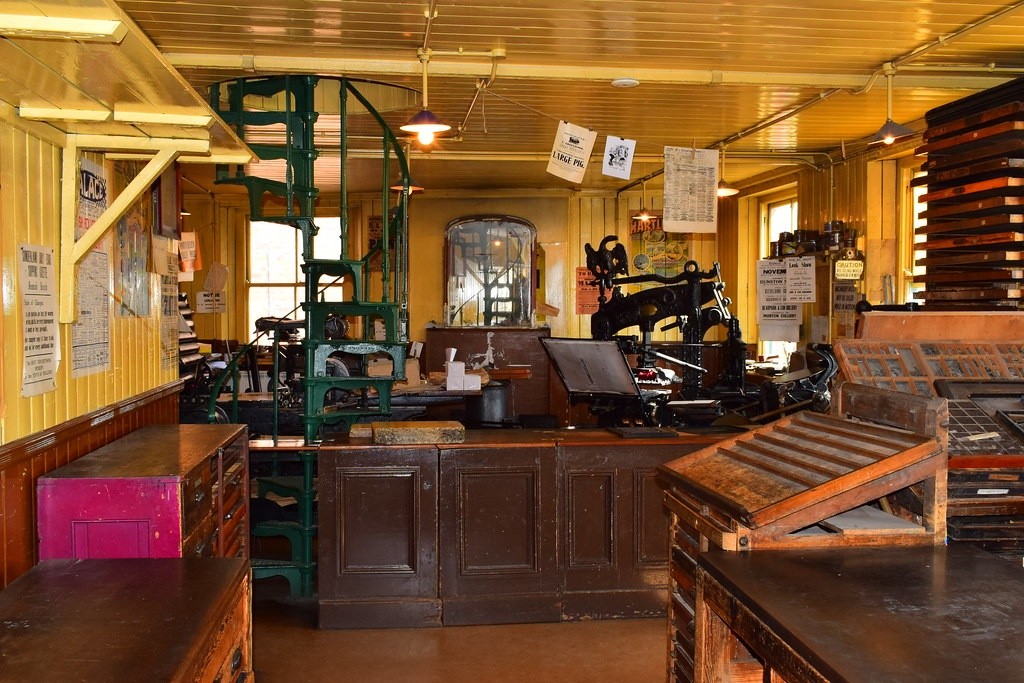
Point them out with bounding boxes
[0,424,255,683]
[318,426,751,629]
[665,311,1024,683]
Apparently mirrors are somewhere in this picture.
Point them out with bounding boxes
[443,212,537,328]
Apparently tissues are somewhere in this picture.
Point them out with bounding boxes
[445,347,465,376]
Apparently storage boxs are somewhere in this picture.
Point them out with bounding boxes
[369,356,420,389]
[446,361,482,390]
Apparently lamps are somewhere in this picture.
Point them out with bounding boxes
[868,73,915,146]
[399,47,452,146]
[718,145,739,197]
[390,139,423,194]
[632,184,657,221]
